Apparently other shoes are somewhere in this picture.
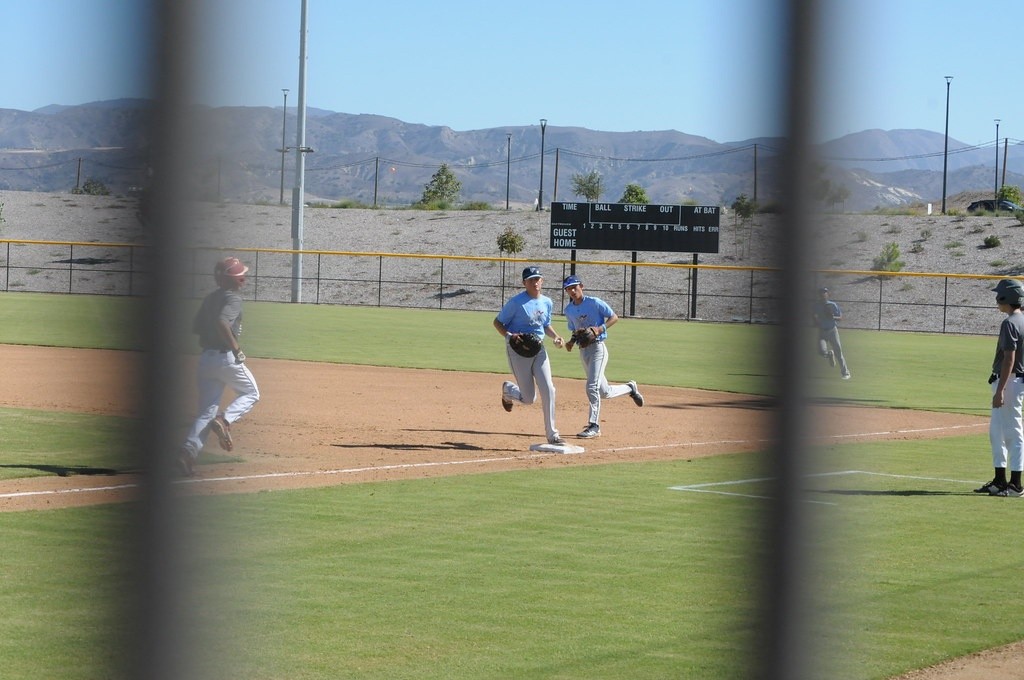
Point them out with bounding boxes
[829,350,836,368]
[841,375,851,380]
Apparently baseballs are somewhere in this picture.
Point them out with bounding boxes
[554,340,564,349]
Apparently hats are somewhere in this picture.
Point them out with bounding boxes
[562,275,586,288]
[522,267,543,281]
[819,287,827,294]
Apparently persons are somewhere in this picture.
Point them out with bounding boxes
[493,266,567,445]
[812,287,851,380]
[176,258,260,478]
[561,275,643,439]
[973,278,1024,497]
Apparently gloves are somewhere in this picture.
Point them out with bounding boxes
[233,348,246,364]
[826,313,834,320]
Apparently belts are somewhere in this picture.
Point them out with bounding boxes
[819,325,835,330]
[988,373,1024,384]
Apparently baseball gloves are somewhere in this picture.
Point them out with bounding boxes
[509,332,543,359]
[570,325,599,349]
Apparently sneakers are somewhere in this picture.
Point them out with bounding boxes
[502,381,513,412]
[176,450,201,477]
[577,423,601,438]
[626,380,644,407]
[550,437,569,444]
[974,480,1006,495]
[211,415,234,451]
[996,483,1024,498]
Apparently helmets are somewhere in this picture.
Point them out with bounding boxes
[215,257,251,278]
[990,279,1024,307]
